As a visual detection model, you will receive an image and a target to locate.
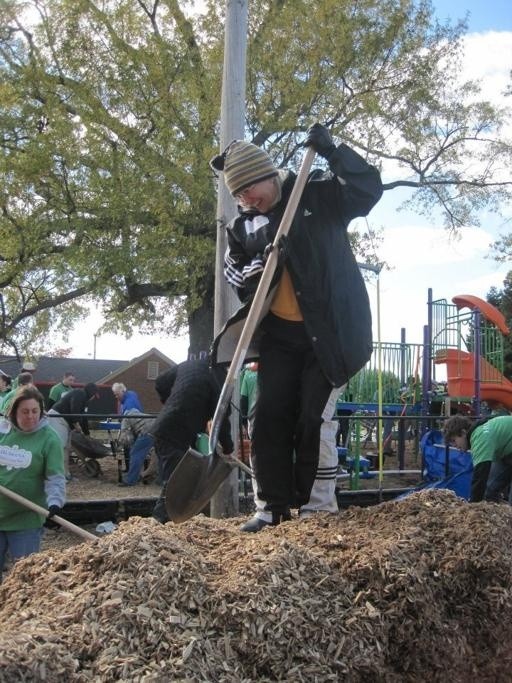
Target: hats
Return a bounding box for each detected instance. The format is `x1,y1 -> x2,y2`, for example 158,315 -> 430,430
209,139 -> 279,198
22,362 -> 36,370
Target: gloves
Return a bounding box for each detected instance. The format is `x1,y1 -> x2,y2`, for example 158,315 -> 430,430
303,123 -> 334,158
42,504 -> 63,531
263,233 -> 290,266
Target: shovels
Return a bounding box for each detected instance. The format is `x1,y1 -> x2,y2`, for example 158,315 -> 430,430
160,147 -> 319,525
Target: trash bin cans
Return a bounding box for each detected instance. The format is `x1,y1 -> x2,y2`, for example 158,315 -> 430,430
421,431 -> 473,500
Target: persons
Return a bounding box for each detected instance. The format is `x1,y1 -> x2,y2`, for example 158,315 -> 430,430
114,407 -> 161,488
1,382 -> 66,584
397,375 -> 415,403
238,359 -> 259,421
111,382 -> 143,475
440,412 -> 512,504
145,351 -> 236,527
45,381 -> 102,484
211,119 -> 384,532
1,371 -> 34,419
333,383 -> 353,448
1,370 -> 12,404
297,379 -> 347,517
45,372 -> 79,431
378,379 -> 398,456
12,361 -> 36,388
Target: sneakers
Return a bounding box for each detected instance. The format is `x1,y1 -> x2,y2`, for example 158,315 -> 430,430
240,504 -> 311,532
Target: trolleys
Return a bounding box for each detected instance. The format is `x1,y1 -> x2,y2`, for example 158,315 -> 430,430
70,430 -> 110,477
